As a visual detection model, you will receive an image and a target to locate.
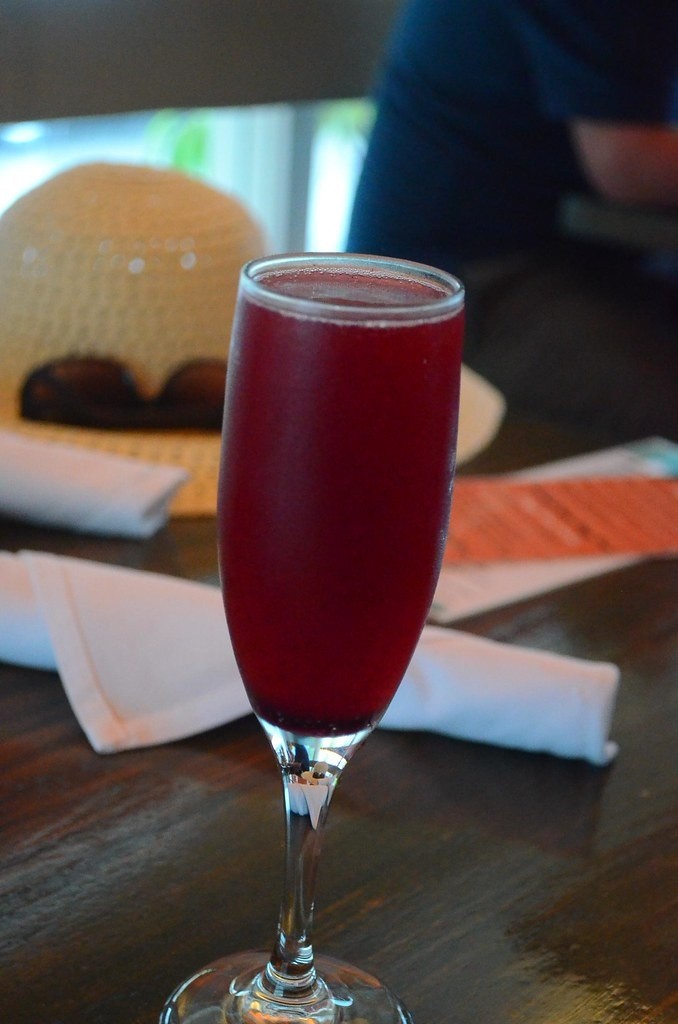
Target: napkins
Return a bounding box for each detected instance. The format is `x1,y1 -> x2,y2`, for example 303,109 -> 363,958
0,419 -> 678,1024
0,429 -> 190,539
0,549 -> 621,765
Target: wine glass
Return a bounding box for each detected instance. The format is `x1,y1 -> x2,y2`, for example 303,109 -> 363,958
155,251 -> 466,1024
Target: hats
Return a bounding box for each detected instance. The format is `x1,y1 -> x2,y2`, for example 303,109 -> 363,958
0,161 -> 507,518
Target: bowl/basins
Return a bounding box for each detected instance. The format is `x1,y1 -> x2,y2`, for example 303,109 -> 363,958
573,119 -> 678,208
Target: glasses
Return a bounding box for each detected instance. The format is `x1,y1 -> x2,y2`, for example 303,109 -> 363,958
20,353 -> 227,434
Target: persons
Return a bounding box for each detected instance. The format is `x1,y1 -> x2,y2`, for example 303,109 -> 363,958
346,0 -> 678,447
